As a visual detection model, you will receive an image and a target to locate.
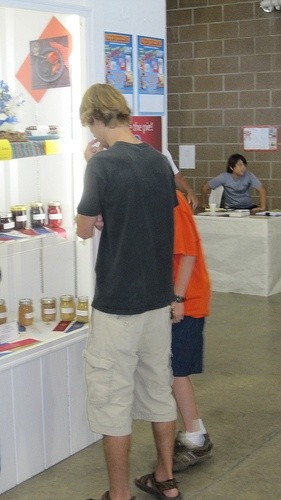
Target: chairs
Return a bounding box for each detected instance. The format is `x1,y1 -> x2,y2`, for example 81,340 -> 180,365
208,185 -> 224,208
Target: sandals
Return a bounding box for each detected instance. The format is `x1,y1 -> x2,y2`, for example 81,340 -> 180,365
135,473 -> 182,500
87,490 -> 137,500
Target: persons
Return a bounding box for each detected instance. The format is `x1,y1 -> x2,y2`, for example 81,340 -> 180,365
202,153 -> 266,212
167,149 -> 198,211
170,188 -> 215,474
75,83 -> 183,500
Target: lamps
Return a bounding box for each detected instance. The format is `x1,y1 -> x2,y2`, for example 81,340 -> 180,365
260,0 -> 281,13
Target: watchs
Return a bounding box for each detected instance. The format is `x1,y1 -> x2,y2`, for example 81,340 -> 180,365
174,295 -> 185,303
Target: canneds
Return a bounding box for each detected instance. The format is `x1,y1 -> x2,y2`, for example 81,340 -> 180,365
9,205 -> 28,229
60,295 -> 75,322
40,297 -> 57,321
30,201 -> 46,227
0,212 -> 15,232
19,298 -> 34,326
0,299 -> 7,324
76,296 -> 88,323
48,202 -> 62,228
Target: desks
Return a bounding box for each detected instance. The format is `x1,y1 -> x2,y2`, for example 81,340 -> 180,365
193,215 -> 281,297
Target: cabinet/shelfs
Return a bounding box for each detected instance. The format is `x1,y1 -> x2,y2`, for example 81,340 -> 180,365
0,139 -> 89,365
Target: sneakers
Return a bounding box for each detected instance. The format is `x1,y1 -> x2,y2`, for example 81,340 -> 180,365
171,432 -> 214,471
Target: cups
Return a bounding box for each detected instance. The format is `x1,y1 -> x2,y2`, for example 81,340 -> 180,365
209,204 -> 216,214
